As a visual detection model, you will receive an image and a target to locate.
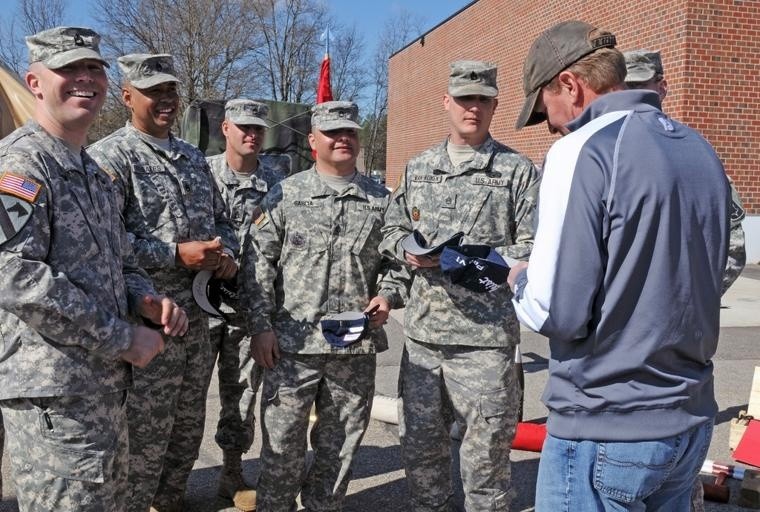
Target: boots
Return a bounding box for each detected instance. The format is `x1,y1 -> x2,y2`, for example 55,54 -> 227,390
215,446 -> 257,512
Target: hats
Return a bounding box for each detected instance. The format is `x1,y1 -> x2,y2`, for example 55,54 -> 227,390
114,52 -> 185,87
513,19 -> 618,130
401,230 -> 466,257
191,267 -> 241,319
309,100 -> 363,130
447,60 -> 501,99
440,243 -> 523,294
620,47 -> 665,82
224,98 -> 271,129
320,311 -> 371,347
23,25 -> 113,71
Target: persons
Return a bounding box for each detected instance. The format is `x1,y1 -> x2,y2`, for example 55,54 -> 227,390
0,25 -> 190,511
380,61 -> 540,511
241,100 -> 414,512
623,46 -> 747,511
81,50 -> 240,512
513,21 -> 732,511
203,98 -> 284,512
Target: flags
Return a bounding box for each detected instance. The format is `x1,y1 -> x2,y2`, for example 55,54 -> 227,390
309,58 -> 333,166
